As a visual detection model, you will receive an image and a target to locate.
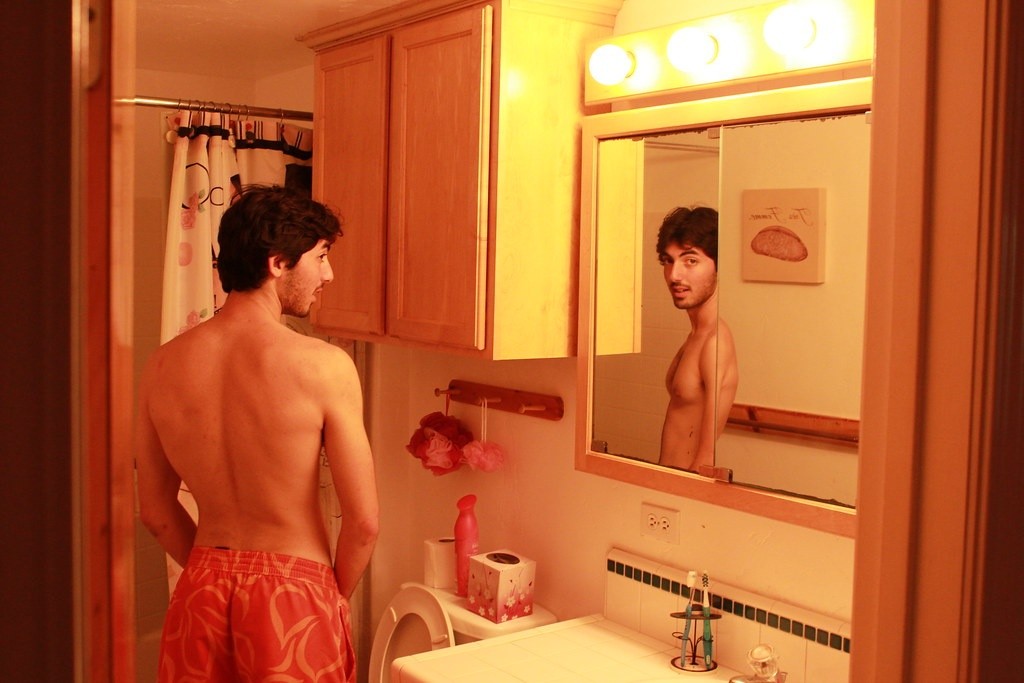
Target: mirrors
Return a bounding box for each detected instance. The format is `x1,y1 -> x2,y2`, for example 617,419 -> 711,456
568,102 -> 875,538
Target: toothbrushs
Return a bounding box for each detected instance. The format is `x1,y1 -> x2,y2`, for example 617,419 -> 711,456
680,570 -> 713,671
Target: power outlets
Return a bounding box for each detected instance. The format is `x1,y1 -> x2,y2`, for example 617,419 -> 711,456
637,501 -> 683,547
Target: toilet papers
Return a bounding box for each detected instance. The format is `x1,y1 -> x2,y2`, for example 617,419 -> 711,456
422,535 -> 460,590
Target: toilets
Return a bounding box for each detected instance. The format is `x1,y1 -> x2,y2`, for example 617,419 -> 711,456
275,584 -> 457,683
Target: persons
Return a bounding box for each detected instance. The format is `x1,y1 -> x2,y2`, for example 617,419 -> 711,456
656,205 -> 736,477
135,184 -> 379,683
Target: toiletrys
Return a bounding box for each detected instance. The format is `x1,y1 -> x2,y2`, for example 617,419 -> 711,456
453,494 -> 481,599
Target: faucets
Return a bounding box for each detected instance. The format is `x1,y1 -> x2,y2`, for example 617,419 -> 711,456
727,643 -> 787,683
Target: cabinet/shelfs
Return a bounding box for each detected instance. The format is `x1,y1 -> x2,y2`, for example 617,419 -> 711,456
434,379 -> 565,424
290,0 -> 578,362
